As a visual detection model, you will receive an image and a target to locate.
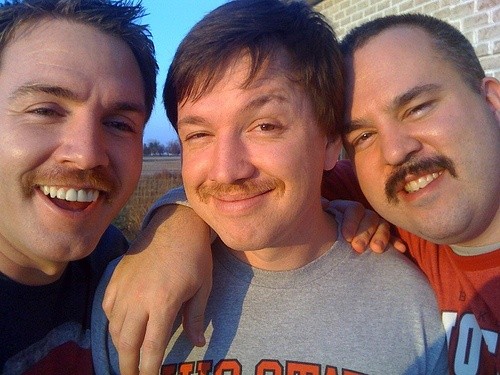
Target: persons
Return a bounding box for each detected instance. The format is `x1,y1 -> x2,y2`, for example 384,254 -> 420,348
0,0 -> 160,375
91,0 -> 452,374
101,12 -> 500,374
